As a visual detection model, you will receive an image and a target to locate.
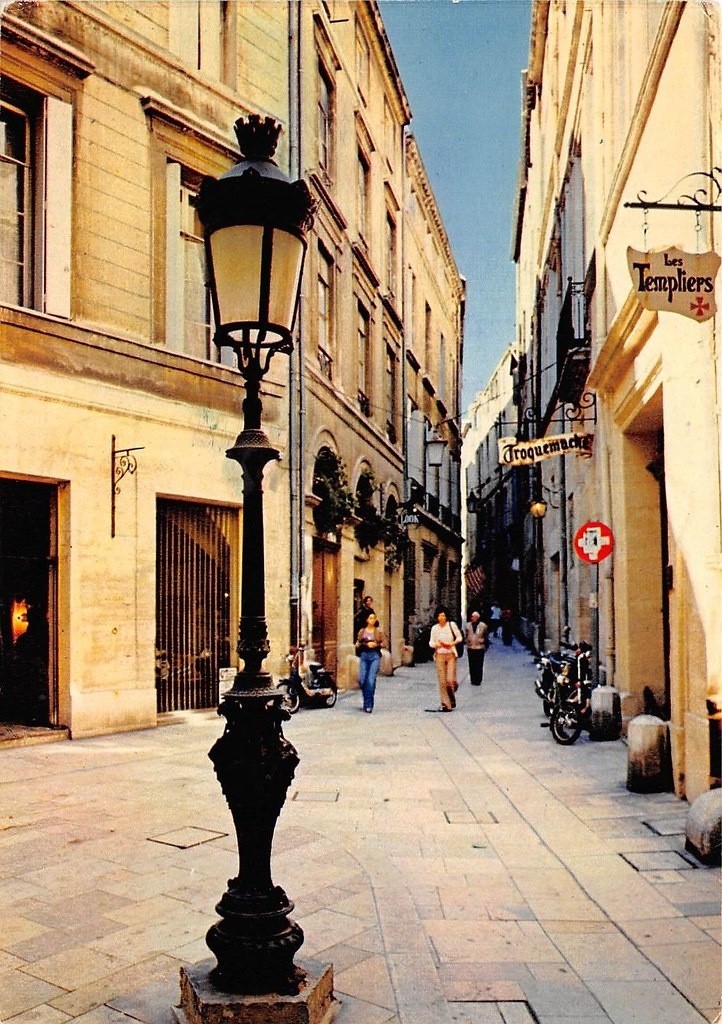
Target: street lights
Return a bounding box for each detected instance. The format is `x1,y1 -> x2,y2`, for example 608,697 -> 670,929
185,109 -> 322,997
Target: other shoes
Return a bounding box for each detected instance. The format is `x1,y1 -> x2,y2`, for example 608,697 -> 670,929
439,706 -> 452,712
366,708 -> 373,713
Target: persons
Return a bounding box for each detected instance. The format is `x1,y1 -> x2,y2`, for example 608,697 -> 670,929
476,599 -> 514,648
429,611 -> 463,712
355,595 -> 380,643
464,611 -> 488,687
355,612 -> 386,713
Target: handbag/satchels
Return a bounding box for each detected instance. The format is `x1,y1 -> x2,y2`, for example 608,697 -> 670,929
449,620 -> 464,658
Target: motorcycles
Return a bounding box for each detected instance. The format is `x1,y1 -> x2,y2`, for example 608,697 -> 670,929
533,622 -> 596,744
271,641 -> 338,715
